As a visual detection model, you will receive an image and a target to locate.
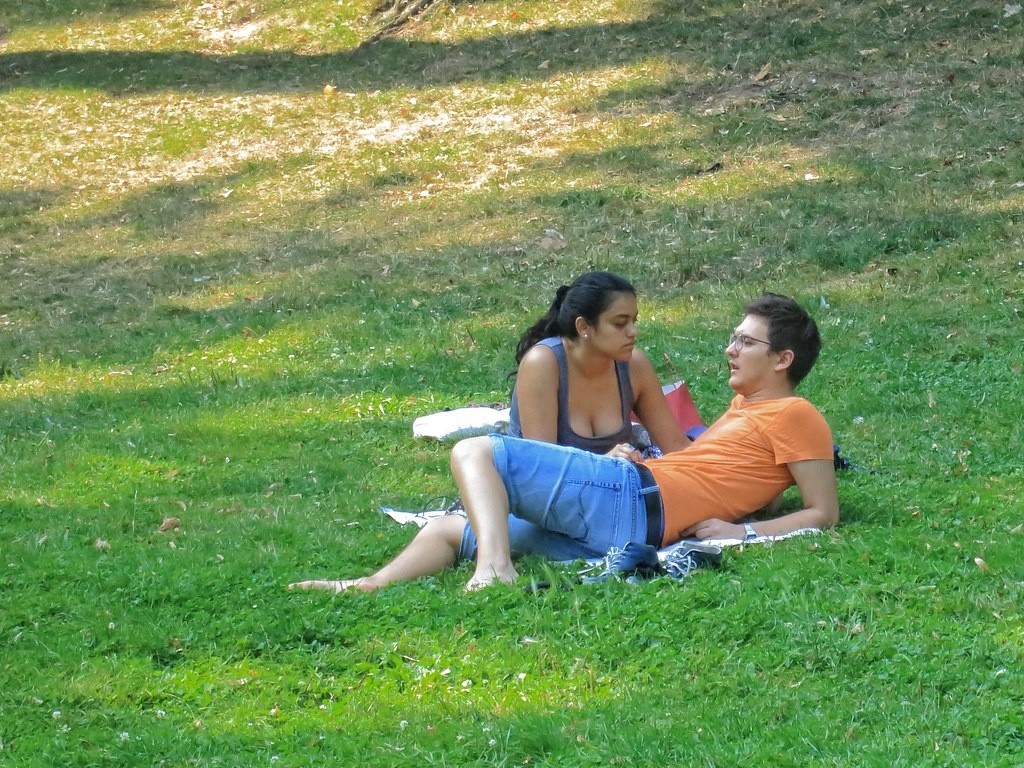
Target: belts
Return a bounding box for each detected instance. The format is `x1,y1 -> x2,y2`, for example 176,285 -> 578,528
630,461 -> 659,552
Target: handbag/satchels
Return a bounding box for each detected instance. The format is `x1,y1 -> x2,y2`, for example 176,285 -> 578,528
630,353 -> 704,432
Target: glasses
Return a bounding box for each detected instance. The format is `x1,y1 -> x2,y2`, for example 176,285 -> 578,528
729,330 -> 774,352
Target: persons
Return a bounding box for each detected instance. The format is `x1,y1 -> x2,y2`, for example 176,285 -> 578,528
509,272 -> 693,464
287,296 -> 840,596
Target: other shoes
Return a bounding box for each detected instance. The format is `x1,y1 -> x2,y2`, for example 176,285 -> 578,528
581,542 -> 658,592
626,541 -> 723,587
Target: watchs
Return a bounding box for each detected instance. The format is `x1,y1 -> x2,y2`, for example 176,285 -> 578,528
744,523 -> 758,541
